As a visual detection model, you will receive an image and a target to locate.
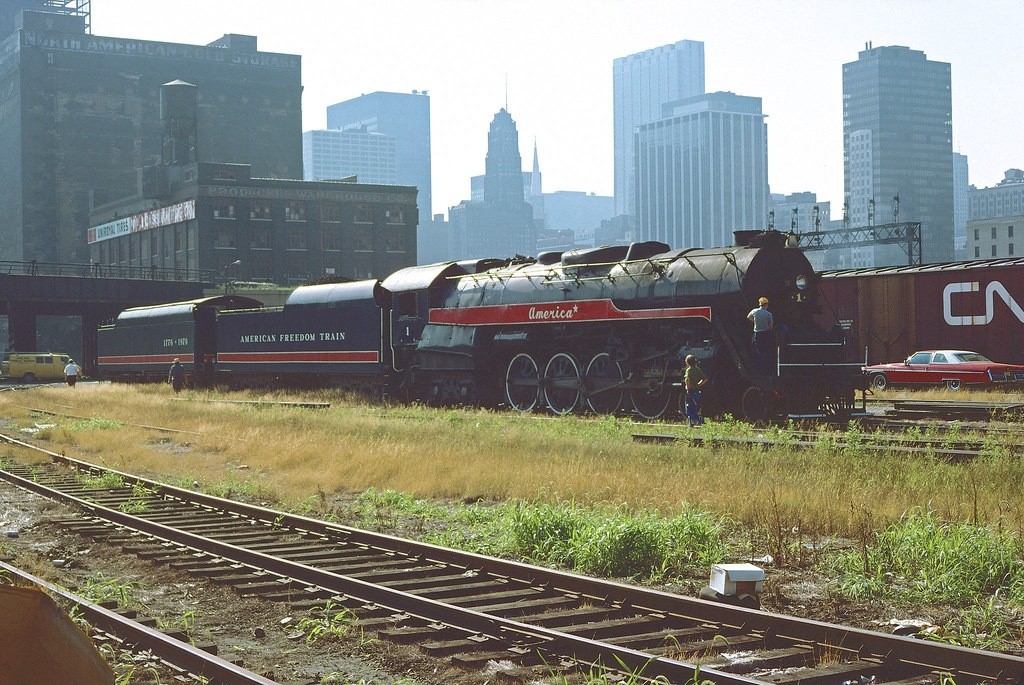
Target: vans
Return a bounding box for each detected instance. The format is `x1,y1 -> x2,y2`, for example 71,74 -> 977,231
9,352 -> 82,384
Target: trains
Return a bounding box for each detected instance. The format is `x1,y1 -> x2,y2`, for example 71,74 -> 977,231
98,231 -> 871,425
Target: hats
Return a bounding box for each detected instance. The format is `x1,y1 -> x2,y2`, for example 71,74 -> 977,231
759,297 -> 769,305
69,359 -> 73,361
174,358 -> 180,362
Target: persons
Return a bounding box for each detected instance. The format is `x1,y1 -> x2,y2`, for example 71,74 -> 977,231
685,355 -> 707,426
168,357 -> 185,392
64,359 -> 79,386
747,297 -> 774,362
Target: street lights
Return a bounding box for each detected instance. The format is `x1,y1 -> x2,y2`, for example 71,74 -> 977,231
225,260 -> 241,296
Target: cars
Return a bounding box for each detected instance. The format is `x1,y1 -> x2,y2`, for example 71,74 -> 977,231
861,350 -> 1024,392
